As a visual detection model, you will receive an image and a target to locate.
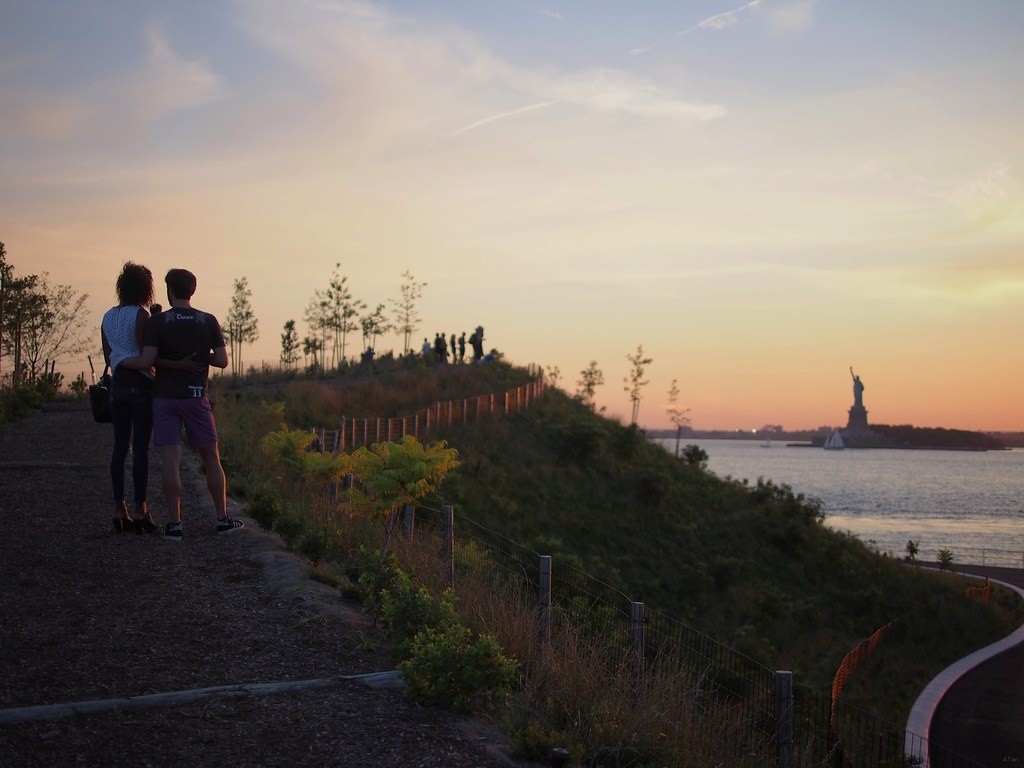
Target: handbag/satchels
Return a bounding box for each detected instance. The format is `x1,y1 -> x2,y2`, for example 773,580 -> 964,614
88,359 -> 113,423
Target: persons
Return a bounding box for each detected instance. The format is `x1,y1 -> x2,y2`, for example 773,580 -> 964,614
120,268 -> 244,542
100,262 -> 164,530
422,326 -> 496,366
849,366 -> 864,406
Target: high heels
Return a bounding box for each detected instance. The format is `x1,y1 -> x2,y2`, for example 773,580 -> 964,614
113,510 -> 134,534
133,511 -> 163,536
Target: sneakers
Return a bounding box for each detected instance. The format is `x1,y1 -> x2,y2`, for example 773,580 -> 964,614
158,521 -> 183,541
216,512 -> 245,536
